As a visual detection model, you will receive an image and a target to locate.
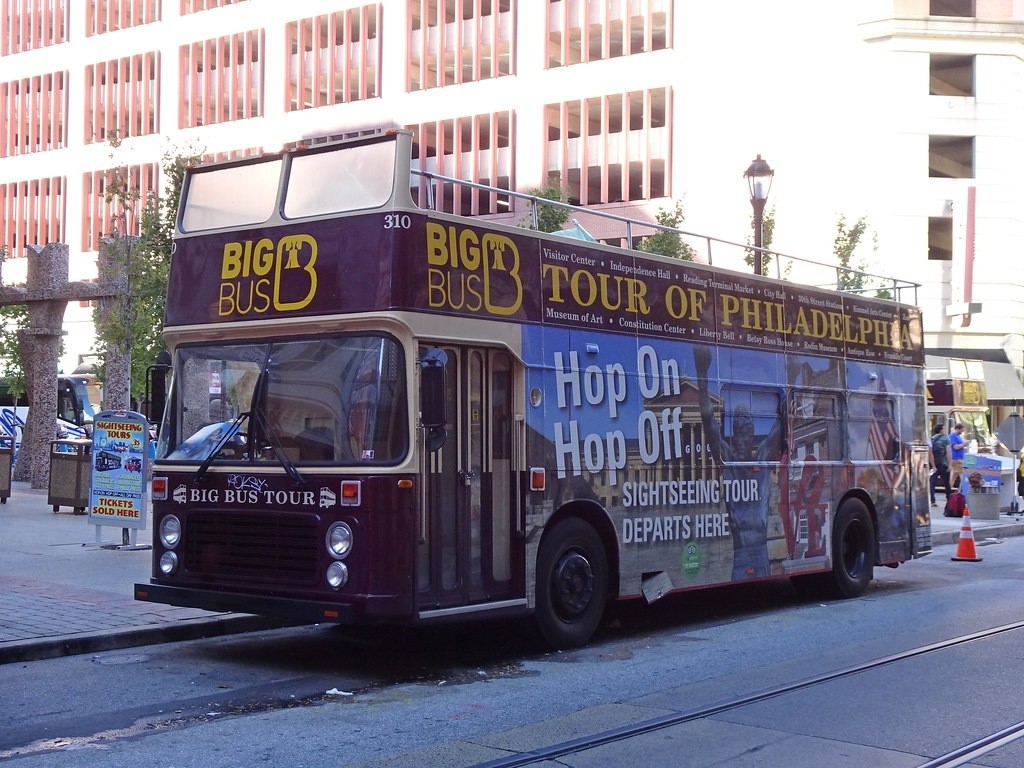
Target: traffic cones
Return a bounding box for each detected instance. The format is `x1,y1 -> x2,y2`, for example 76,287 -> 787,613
950,508 -> 983,562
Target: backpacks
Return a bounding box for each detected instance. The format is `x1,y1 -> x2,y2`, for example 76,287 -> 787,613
930,434 -> 947,467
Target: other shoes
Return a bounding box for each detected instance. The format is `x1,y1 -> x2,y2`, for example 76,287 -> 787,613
932,503 -> 937,507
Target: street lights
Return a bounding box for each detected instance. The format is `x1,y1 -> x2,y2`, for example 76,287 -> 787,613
744,155 -> 775,274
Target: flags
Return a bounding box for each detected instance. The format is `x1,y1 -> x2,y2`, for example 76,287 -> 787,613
869,372 -> 905,496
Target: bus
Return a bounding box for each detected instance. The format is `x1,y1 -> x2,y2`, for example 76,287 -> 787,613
131,122 -> 932,652
0,376 -> 96,460
924,358 -> 995,487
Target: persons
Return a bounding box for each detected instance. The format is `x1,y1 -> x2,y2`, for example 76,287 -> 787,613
930,424 -> 951,507
948,423 -> 970,486
1018,447 -> 1024,516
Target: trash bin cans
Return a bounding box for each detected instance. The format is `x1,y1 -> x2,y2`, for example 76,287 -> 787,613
963,453 -> 1001,520
48,438 -> 92,515
0,435 -> 16,504
